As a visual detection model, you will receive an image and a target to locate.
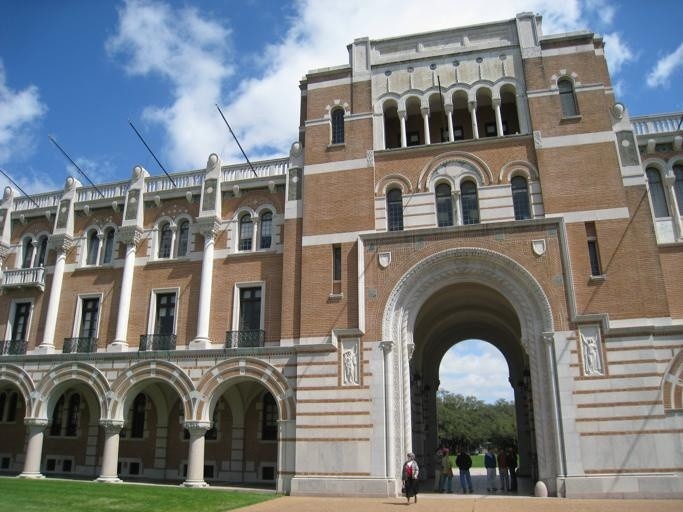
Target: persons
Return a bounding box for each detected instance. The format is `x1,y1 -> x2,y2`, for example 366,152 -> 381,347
432,446 -> 518,493
402,452 -> 420,504
